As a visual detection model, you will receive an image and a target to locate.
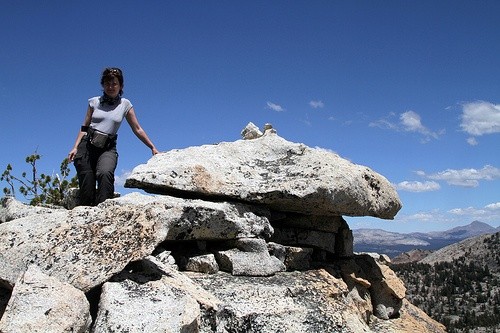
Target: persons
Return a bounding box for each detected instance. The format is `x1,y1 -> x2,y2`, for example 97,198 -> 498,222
68,68 -> 160,206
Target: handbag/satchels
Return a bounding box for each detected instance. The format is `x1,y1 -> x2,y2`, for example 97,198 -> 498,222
81,126 -> 117,149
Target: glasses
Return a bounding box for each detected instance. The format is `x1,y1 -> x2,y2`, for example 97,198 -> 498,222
103,70 -> 121,76
